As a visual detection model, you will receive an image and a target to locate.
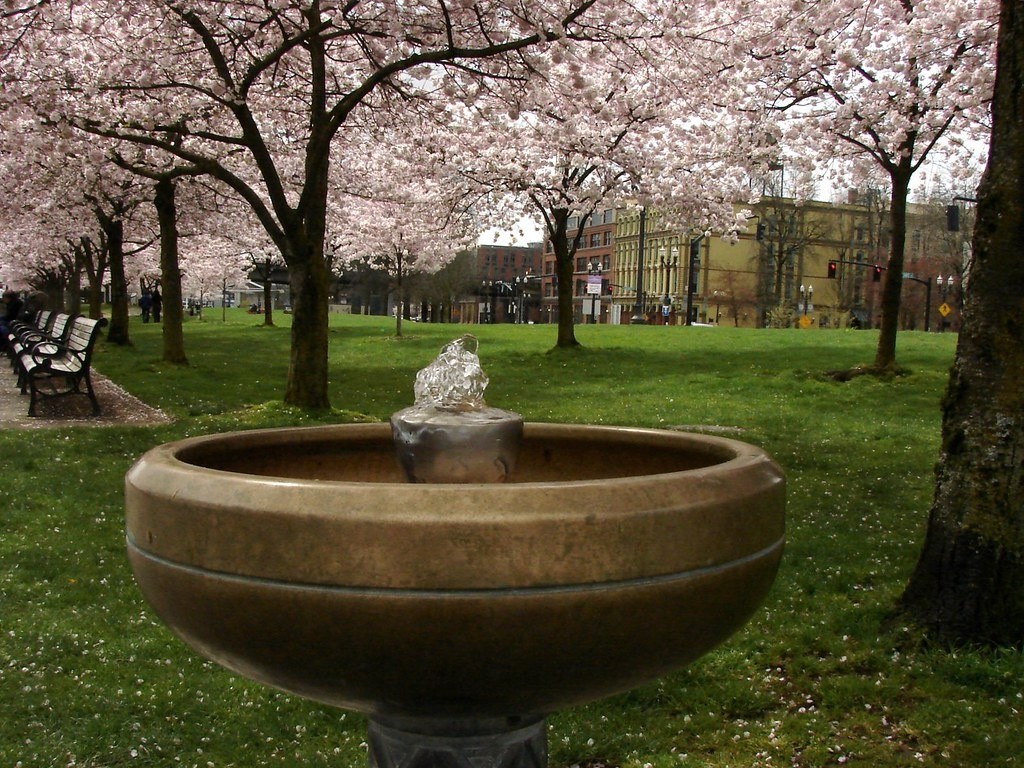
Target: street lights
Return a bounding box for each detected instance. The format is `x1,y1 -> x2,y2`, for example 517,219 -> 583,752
515,275 -> 528,324
937,273 -> 954,334
800,284 -> 814,316
587,261 -> 603,323
769,162 -> 784,306
714,290 -> 725,327
659,244 -> 679,326
482,280 -> 492,324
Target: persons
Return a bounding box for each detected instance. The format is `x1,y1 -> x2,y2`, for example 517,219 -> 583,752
0,291 -> 23,333
138,289 -> 162,323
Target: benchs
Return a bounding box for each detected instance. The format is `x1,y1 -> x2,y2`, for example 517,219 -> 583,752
5,309 -> 111,420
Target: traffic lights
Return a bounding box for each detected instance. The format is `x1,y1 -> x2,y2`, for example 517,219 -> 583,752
827,262 -> 836,279
872,265 -> 882,283
607,286 -> 612,295
755,223 -> 767,241
